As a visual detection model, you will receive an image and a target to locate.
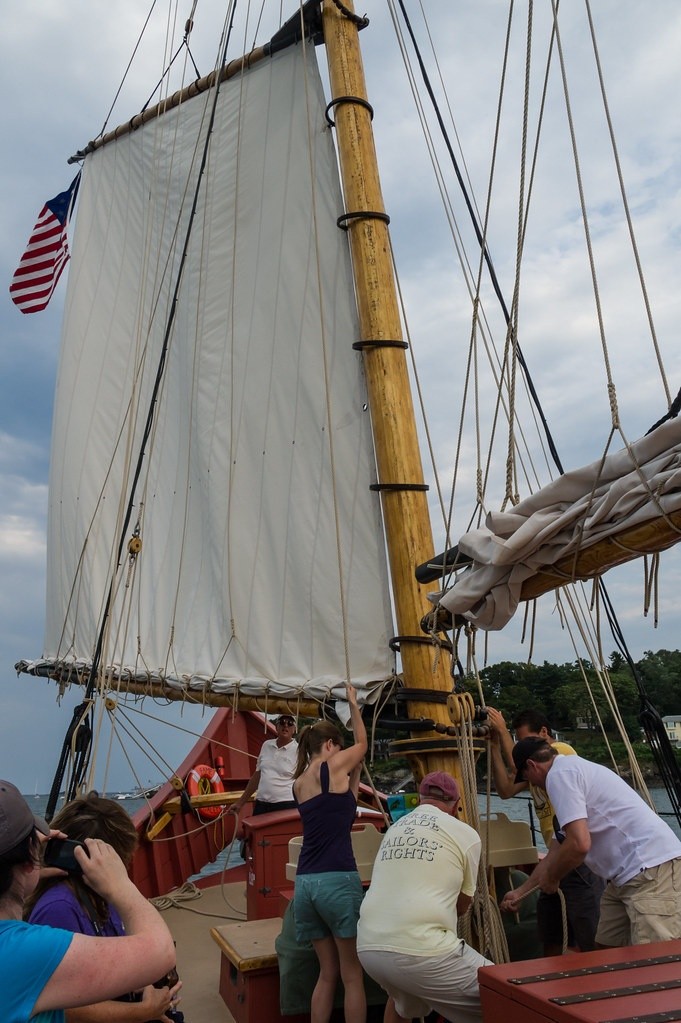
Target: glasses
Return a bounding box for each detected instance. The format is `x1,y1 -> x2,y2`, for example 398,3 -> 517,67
278,719 -> 295,726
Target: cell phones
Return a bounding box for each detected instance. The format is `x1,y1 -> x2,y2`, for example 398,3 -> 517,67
42,837 -> 94,876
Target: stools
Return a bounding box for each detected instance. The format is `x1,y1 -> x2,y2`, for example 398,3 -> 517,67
210,915 -> 317,1022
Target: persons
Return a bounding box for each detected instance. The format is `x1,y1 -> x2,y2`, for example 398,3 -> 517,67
23,790 -> 177,1022
357,771 -> 497,1023
511,734 -> 678,950
227,715 -> 299,900
479,704 -> 605,960
288,681 -> 371,1023
0,781 -> 176,1022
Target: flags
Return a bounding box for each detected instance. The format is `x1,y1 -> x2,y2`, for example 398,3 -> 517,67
7,167 -> 81,312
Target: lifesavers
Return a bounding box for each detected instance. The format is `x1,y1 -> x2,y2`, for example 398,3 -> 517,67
187,765 -> 225,819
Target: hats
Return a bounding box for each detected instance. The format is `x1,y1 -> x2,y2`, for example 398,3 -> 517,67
277,714 -> 297,722
0,779 -> 50,856
420,772 -> 459,801
513,736 -> 548,785
